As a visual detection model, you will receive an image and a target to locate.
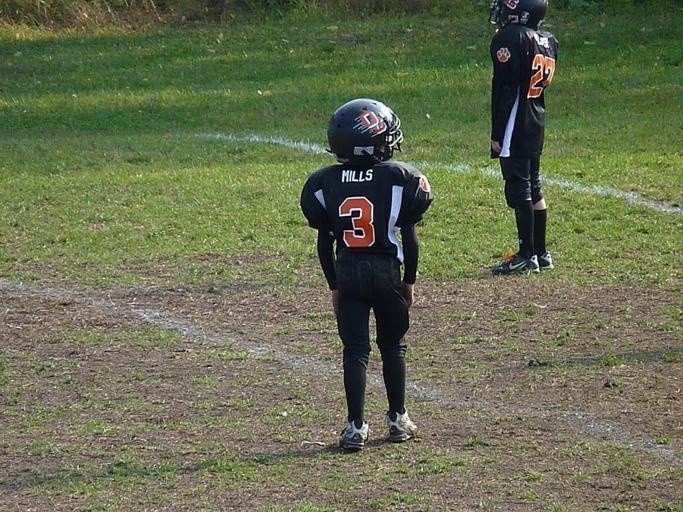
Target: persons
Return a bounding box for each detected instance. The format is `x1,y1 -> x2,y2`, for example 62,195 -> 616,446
299,98 -> 437,450
486,1 -> 560,274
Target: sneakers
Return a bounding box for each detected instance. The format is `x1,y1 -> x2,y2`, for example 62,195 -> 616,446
492,253 -> 540,275
538,251 -> 555,269
339,419 -> 370,450
387,407 -> 418,442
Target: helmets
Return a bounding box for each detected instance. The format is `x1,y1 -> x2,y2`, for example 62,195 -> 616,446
327,99 -> 393,163
494,0 -> 549,28
